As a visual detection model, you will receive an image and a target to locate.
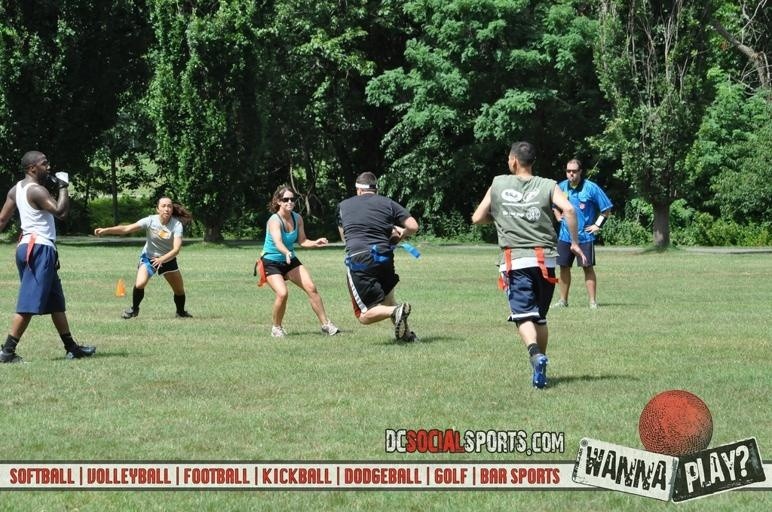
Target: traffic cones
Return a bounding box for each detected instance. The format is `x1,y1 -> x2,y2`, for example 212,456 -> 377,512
113,277 -> 129,298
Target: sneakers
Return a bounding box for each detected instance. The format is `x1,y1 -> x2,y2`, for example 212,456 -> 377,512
530,353 -> 547,389
272,326 -> 288,337
549,301 -> 568,309
322,323 -> 340,336
590,303 -> 598,310
175,312 -> 193,318
66,346 -> 96,359
121,307 -> 139,318
392,303 -> 416,343
0,350 -> 23,363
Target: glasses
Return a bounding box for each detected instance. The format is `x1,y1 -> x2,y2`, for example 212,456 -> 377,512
281,198 -> 296,202
567,170 -> 577,173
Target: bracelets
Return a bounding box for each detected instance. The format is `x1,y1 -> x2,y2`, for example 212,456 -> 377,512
595,214 -> 607,228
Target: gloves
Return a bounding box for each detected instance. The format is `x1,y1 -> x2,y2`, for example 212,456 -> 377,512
50,172 -> 69,189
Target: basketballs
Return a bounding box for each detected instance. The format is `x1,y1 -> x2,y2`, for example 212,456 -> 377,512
639,390 -> 713,457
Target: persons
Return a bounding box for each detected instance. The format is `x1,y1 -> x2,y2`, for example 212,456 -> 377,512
337,172 -> 419,343
254,185 -> 339,337
0,151 -> 96,361
472,143 -> 587,389
552,159 -> 613,308
94,196 -> 193,318
